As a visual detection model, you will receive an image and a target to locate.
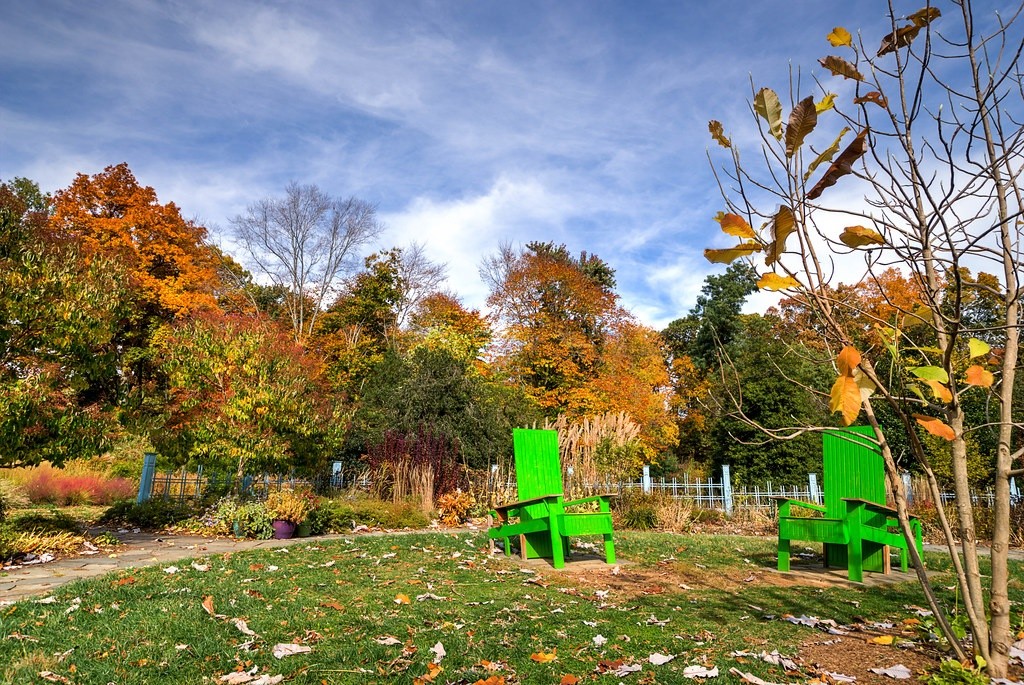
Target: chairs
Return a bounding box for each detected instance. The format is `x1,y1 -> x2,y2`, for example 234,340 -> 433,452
769,425 -> 924,583
487,428 -> 619,569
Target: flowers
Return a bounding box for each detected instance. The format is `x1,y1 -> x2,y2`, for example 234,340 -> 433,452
266,485 -> 321,526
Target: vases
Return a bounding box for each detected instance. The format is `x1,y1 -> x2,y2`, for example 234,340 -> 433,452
271,520 -> 298,541
293,518 -> 313,538
